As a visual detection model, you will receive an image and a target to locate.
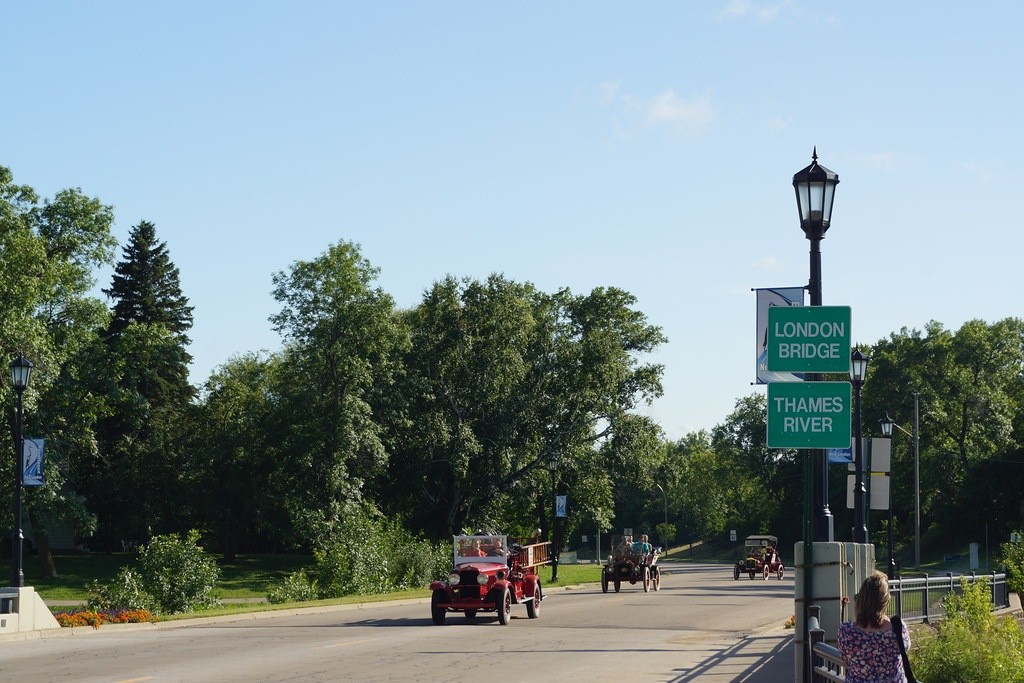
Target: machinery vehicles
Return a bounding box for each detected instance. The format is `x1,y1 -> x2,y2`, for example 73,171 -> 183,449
428,529 -> 552,625
600,534 -> 662,593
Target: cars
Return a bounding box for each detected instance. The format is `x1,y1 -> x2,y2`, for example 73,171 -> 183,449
732,535 -> 786,581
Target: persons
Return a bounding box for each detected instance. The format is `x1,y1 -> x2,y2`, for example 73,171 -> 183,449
836,571 -> 911,683
487,538 -> 509,556
643,534 -> 652,553
750,542 -> 777,564
633,535 -> 649,555
627,535 -> 635,545
464,539 -> 486,558
616,535 -> 633,555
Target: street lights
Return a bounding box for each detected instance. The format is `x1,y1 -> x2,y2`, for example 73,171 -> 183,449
791,146 -> 844,683
656,482 -> 667,560
877,412 -> 896,596
547,455 -> 561,583
846,340 -> 872,545
6,350 -> 34,589
